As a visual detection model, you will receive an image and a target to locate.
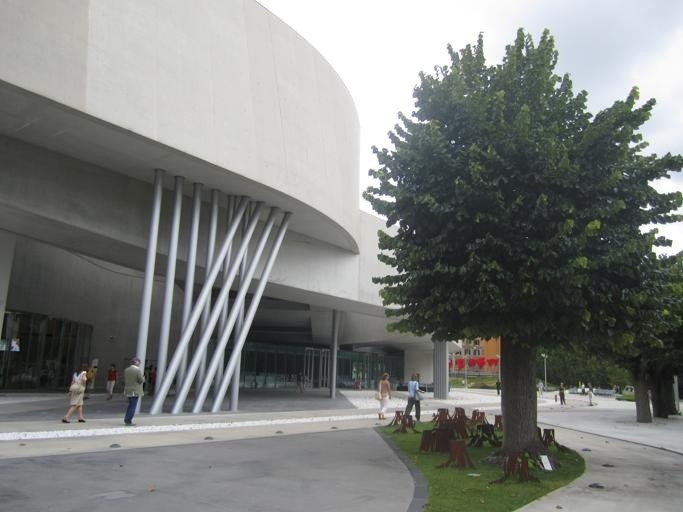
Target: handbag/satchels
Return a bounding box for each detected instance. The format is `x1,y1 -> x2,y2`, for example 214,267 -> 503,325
69,383 -> 84,392
415,392 -> 424,401
375,392 -> 381,399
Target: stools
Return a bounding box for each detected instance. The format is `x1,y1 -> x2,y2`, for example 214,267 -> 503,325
383,405 -> 562,484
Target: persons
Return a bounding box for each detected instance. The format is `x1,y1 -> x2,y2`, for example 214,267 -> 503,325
588,389 -> 593,406
105,364 -> 116,400
496,379 -> 501,394
376,373 -> 392,419
559,382 -> 567,407
62,364 -> 89,423
403,374 -> 420,421
578,381 -> 592,394
295,371 -> 304,393
537,379 -> 544,398
123,357 -> 145,425
613,384 -> 621,394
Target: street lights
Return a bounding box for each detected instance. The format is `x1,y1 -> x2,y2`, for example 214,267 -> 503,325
464,343 -> 479,390
540,352 -> 548,390
